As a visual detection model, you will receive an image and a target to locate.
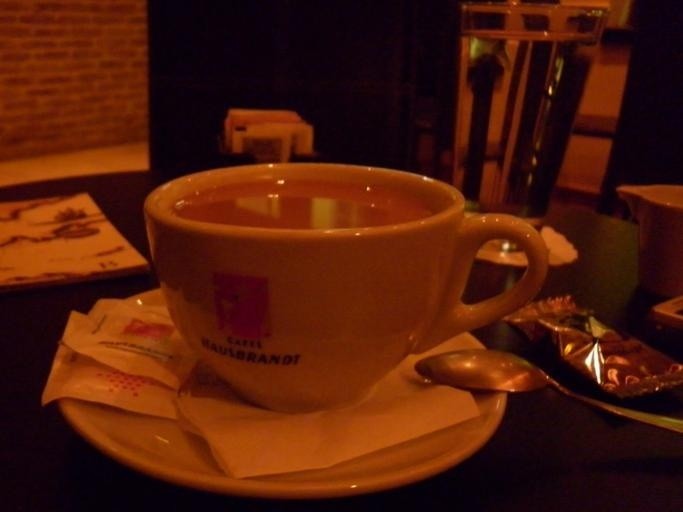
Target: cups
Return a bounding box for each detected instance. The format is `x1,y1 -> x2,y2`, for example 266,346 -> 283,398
617,183 -> 683,303
140,161 -> 548,404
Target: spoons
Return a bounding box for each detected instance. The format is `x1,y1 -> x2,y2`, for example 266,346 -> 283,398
414,349 -> 682,434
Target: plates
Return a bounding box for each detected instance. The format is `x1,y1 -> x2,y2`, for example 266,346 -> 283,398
63,287 -> 506,494
